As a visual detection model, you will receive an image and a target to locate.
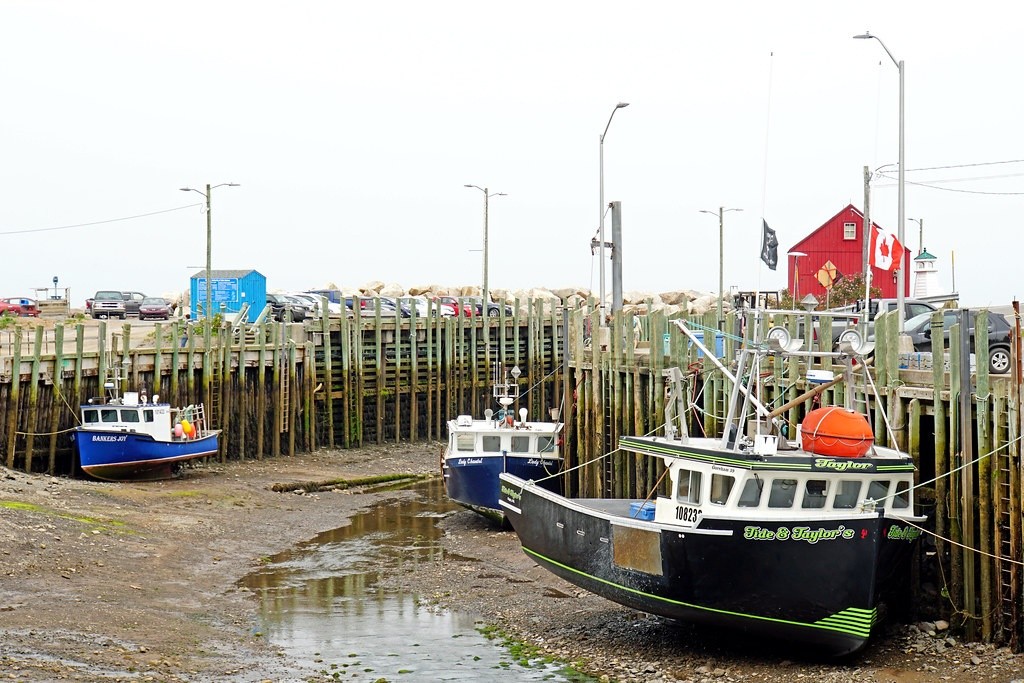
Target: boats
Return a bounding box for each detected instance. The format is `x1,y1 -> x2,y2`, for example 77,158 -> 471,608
439,360 -> 569,529
498,317 -> 934,671
61,355 -> 224,483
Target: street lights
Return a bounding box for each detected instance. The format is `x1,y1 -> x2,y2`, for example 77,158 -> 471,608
699,206 -> 745,317
178,181 -> 242,330
598,100 -> 630,325
852,30 -> 908,345
463,181 -> 507,313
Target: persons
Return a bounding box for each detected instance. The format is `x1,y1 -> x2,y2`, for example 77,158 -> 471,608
606,310 -> 642,350
180,314 -> 193,347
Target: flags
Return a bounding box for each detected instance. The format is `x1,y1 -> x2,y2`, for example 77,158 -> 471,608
760,218 -> 779,271
868,224 -> 904,271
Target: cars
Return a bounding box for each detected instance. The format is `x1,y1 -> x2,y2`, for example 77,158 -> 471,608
90,290 -> 127,320
137,297 -> 171,321
122,291 -> 152,314
283,289 -> 480,319
265,294 -> 306,323
460,296 -> 513,318
0,297 -> 41,318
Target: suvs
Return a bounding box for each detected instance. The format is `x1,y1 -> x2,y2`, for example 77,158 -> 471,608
866,308 -> 1013,375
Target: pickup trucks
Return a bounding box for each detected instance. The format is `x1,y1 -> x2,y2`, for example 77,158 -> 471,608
806,293 -> 937,366
